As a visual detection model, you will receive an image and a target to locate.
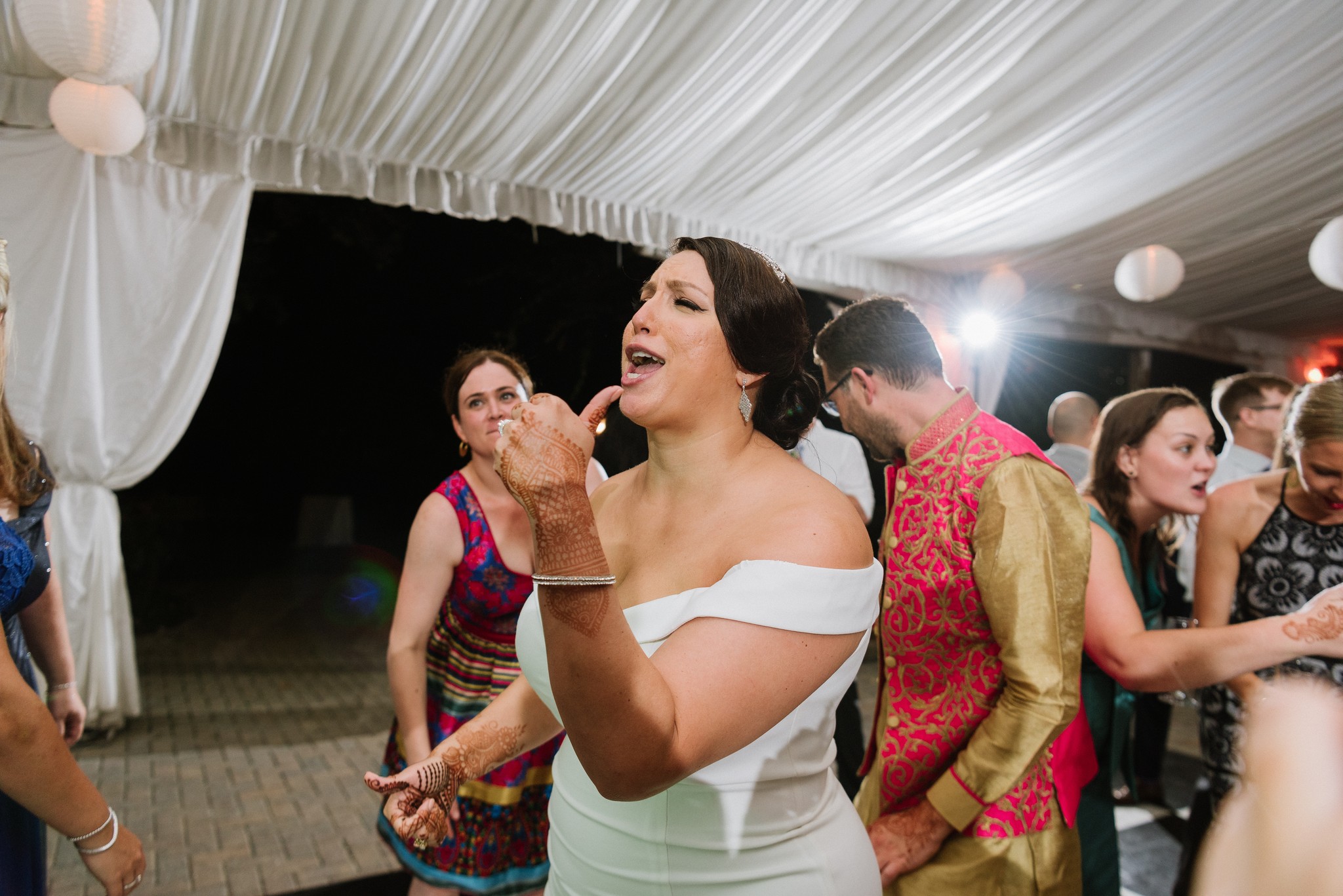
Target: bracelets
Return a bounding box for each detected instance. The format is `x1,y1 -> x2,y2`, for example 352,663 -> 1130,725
529,574 -> 617,586
67,806 -> 114,842
78,811 -> 119,855
47,681 -> 78,692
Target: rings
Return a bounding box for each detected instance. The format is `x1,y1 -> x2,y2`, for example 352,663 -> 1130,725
413,836 -> 428,852
122,874 -> 142,890
498,417 -> 515,436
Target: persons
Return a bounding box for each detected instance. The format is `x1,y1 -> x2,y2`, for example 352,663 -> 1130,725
744,290 -> 1342,895
0,236 -> 86,896
374,345 -> 610,896
0,512 -> 150,896
362,236 -> 886,896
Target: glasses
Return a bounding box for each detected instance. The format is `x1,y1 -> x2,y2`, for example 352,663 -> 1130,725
820,368 -> 874,417
